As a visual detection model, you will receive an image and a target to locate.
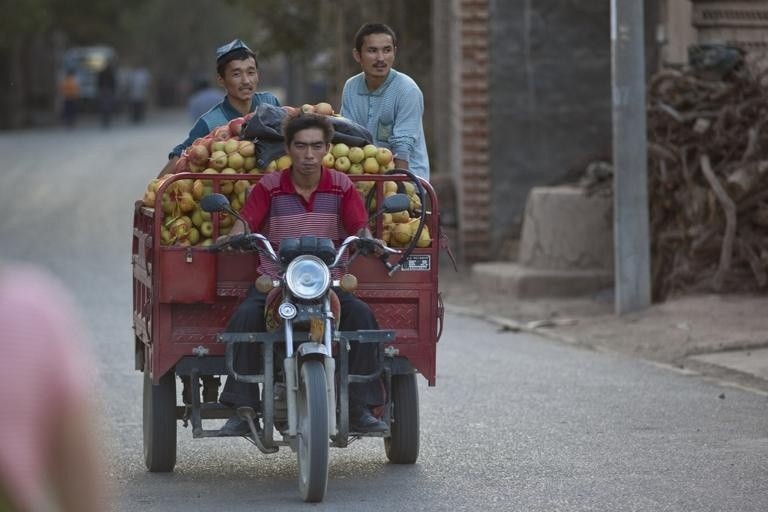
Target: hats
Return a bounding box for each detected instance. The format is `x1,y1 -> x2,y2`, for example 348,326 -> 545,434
216,39 -> 253,60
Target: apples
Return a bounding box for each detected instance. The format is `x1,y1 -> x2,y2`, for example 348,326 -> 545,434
143,102 -> 431,247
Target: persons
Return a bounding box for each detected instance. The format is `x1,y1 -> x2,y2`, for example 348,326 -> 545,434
217,112 -> 390,435
340,21 -> 430,202
55,52 -> 148,130
156,37 -> 284,179
0,264 -> 112,511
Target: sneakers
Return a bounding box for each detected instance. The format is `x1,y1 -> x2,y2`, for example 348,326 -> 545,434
220,415 -> 259,435
350,406 -> 388,432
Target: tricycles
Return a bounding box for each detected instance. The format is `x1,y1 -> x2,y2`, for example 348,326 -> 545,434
128,169 -> 446,506
